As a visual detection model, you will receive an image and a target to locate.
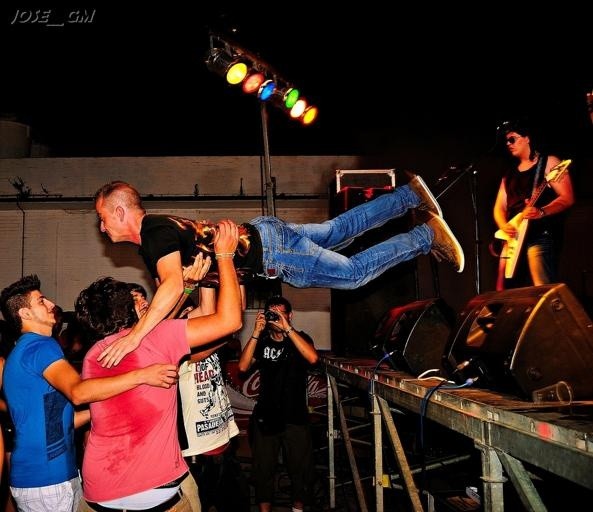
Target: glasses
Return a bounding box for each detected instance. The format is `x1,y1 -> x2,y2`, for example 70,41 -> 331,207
507,135 -> 521,143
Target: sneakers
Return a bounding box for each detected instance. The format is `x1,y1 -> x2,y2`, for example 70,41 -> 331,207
409,175 -> 443,218
428,217 -> 466,273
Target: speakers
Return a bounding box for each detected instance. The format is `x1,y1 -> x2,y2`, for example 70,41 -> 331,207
331,189 -> 420,360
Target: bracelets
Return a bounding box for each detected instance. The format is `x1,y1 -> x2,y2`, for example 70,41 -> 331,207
285,327 -> 293,335
251,335 -> 260,340
537,206 -> 545,217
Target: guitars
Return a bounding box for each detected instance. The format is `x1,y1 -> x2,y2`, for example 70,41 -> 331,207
495,159 -> 570,280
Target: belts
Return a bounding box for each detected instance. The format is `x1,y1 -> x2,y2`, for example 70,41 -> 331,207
87,488 -> 182,512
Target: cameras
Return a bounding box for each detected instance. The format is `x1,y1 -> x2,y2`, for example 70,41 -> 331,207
264,311 -> 279,322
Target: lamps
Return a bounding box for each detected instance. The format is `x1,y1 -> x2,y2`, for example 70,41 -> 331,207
202,36 -> 319,127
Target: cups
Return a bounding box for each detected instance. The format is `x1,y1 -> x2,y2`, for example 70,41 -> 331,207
532,380 -> 573,406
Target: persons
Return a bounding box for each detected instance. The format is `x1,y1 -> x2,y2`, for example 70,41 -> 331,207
238,295 -> 319,511
0,251 -> 247,512
492,118 -> 575,288
93,172 -> 467,371
73,218 -> 245,512
